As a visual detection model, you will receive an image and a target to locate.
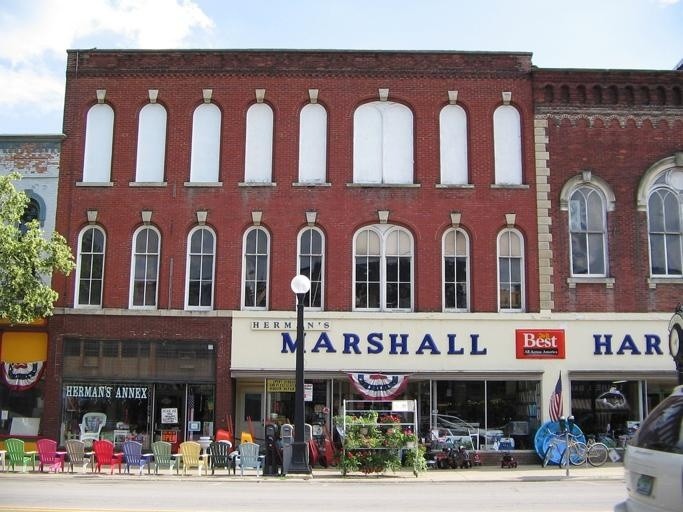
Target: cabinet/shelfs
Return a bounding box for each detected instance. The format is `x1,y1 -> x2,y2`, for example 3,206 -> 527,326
334,400 -> 420,478
514,381 -> 540,449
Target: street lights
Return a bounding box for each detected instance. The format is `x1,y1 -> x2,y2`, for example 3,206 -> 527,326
287,275 -> 311,474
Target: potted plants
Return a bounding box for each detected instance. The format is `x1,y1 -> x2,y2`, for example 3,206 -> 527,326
333,412 -> 428,479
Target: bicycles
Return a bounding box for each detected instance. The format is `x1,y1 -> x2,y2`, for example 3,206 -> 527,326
559,433 -> 608,469
541,424 -> 588,468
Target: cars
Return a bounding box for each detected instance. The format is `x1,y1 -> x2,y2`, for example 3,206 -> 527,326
419,413 -> 504,451
614,385 -> 682,511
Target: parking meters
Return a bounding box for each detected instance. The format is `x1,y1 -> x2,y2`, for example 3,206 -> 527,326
558,414 -> 574,477
667,303 -> 682,386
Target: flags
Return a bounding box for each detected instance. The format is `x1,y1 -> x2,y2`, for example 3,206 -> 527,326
549,376 -> 562,422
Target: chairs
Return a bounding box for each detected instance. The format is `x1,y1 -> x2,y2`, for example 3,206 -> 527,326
0,434 -> 265,478
77,412 -> 107,442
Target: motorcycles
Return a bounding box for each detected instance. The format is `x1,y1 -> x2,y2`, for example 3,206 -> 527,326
421,438 -> 481,469
